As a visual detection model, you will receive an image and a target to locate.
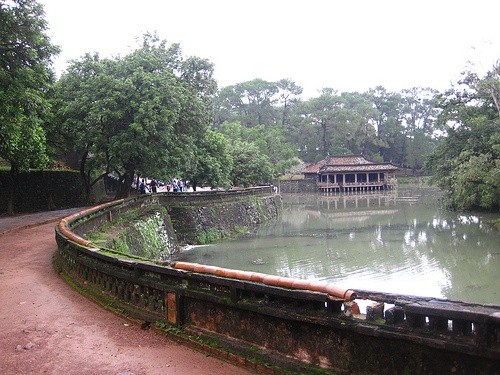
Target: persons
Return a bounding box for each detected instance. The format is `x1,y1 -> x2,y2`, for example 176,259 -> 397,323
140,178 -> 190,193
